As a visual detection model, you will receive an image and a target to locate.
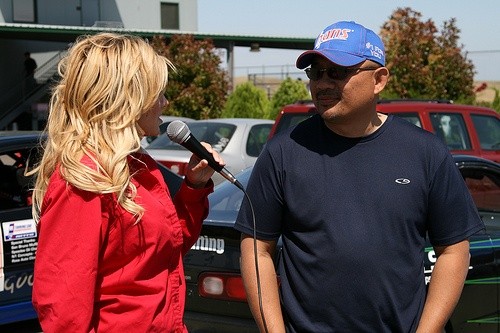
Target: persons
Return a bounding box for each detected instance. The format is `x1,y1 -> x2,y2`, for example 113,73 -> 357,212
232,21 -> 484,333
24,31 -> 226,333
21,51 -> 37,93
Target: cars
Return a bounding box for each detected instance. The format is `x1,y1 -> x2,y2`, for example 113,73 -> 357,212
0,129 -> 51,326
140,118 -> 275,186
182,155 -> 500,333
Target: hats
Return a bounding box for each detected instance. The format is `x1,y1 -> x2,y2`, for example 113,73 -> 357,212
296,20 -> 385,69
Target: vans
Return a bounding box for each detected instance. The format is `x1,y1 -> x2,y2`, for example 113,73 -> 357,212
264,97 -> 500,212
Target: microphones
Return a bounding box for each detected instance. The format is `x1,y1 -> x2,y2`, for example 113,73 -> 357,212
167,120 -> 244,191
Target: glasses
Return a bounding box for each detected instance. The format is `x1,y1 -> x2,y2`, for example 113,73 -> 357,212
304,67 -> 376,82
